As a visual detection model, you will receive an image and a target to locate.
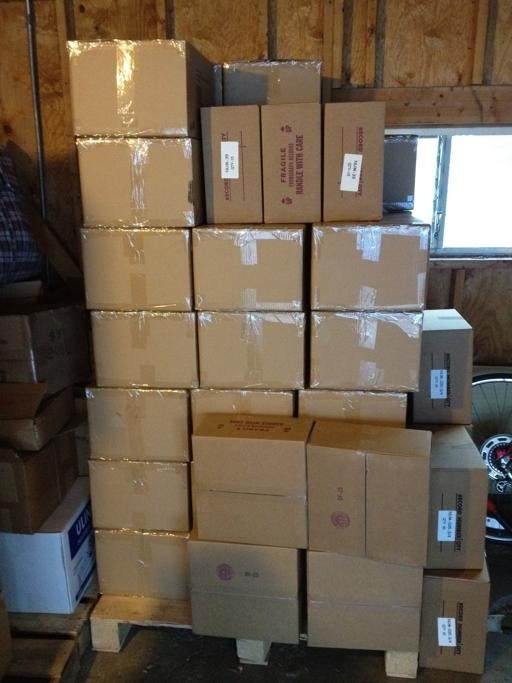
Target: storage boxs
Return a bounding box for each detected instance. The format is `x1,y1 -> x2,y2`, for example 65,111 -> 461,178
1,33 -> 493,675
